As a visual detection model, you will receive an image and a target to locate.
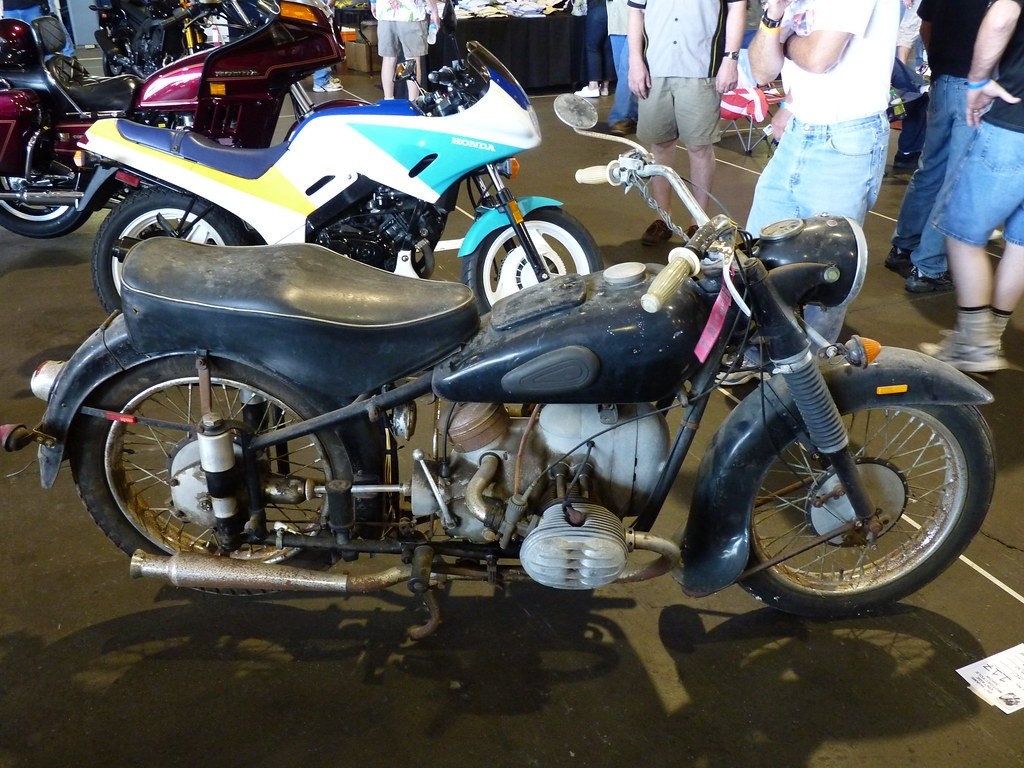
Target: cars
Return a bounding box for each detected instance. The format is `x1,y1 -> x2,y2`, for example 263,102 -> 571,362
0,0 -> 76,92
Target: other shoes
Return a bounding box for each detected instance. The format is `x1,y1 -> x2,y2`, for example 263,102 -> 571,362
611,120 -> 632,135
641,220 -> 673,245
683,225 -> 699,237
893,152 -> 921,167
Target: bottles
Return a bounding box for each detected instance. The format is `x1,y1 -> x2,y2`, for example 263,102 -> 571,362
890,90 -> 907,119
427,22 -> 437,45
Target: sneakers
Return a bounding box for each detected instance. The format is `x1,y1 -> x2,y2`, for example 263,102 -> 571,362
919,335 -> 999,372
574,86 -> 599,97
905,265 -> 953,293
885,247 -> 914,274
313,80 -> 343,92
599,87 -> 609,96
329,76 -> 340,84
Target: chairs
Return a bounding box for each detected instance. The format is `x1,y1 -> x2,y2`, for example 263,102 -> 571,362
720,30 -> 785,156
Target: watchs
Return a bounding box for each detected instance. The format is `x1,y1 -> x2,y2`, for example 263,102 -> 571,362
724,51 -> 739,60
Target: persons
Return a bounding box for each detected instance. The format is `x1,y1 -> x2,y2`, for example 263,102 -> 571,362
2,0 -> 76,62
370,0 -> 463,99
716,0 -> 900,386
573,0 -> 748,248
313,0 -> 343,93
883,0 -> 1024,382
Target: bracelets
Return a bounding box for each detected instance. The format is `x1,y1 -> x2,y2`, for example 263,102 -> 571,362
968,78 -> 990,89
761,8 -> 784,28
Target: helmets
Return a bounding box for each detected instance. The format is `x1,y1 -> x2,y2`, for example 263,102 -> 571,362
0,19 -> 40,69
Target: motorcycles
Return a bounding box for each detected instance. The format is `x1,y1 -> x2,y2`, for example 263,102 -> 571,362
0,0 -> 374,239
0,93 -> 998,621
73,0 -> 606,317
